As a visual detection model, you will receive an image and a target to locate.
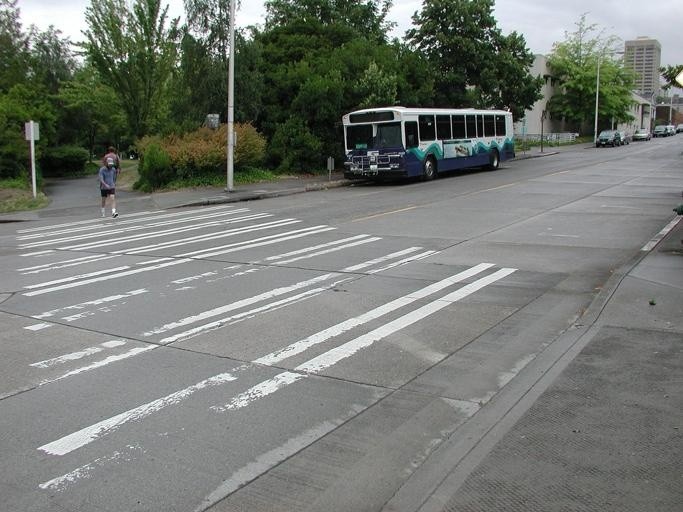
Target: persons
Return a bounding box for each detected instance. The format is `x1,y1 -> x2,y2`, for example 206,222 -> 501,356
102,145 -> 122,187
98,158 -> 118,218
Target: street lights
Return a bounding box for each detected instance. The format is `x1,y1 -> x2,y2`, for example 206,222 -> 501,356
593,48 -> 626,149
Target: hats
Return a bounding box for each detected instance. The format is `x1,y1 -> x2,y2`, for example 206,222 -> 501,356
107,158 -> 113,164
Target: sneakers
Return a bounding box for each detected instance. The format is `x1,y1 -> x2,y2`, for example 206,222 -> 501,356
101,212 -> 118,218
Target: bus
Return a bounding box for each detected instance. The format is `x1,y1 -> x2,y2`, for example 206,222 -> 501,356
341,104 -> 517,183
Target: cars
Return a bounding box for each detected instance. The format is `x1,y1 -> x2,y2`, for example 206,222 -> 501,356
594,124 -> 682,148
